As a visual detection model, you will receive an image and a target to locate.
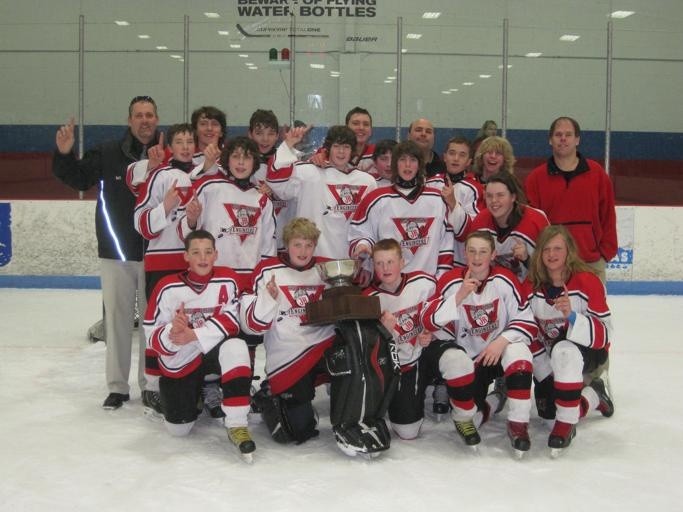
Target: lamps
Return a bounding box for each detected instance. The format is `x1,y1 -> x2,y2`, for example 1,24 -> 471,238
269,47 -> 289,62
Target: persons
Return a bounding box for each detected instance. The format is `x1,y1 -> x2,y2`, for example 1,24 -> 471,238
51,92 -> 623,463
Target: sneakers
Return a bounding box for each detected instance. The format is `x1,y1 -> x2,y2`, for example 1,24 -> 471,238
433,384 -> 448,414
506,421 -> 530,451
452,419 -> 480,445
103,393 -> 129,409
548,421 -> 576,448
143,389 -> 162,415
226,426 -> 255,453
590,378 -> 613,417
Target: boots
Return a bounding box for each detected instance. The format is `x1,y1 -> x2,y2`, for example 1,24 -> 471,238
203,380 -> 225,417
